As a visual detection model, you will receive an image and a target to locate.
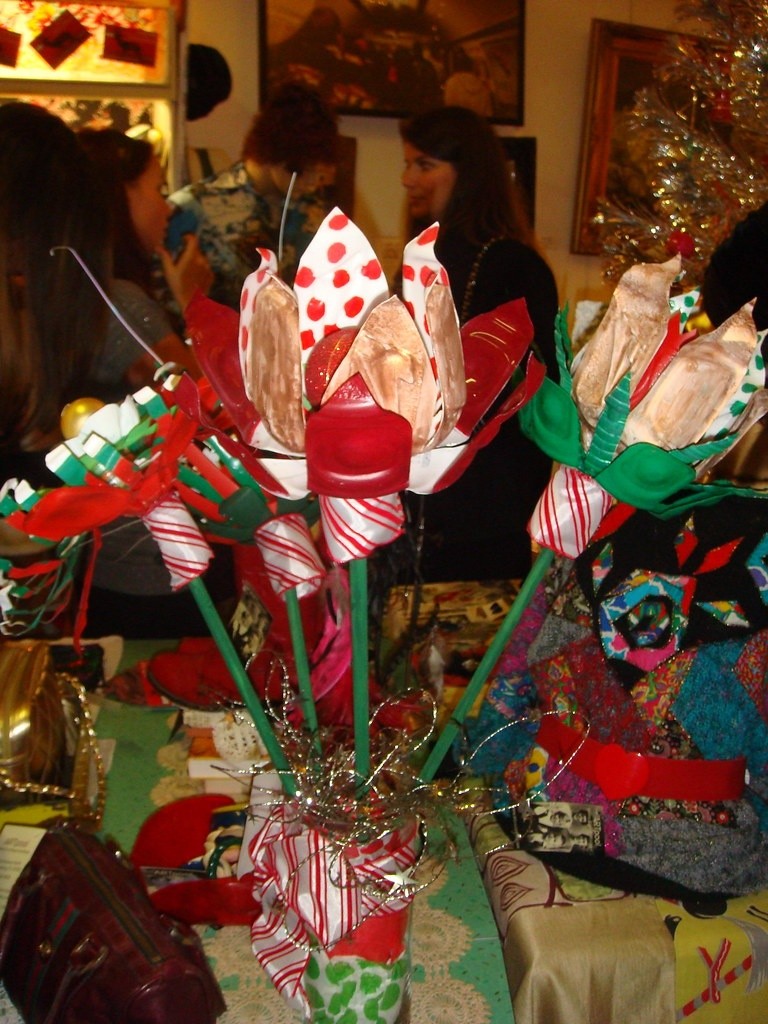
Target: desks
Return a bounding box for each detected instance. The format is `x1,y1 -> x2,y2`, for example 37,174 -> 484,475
374,581 -> 768,1024
4,634 -> 518,1024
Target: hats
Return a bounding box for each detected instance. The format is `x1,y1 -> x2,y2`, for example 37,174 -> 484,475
243,79 -> 350,165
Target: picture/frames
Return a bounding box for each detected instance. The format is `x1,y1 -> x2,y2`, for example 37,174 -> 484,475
567,13 -> 734,258
405,136 -> 537,249
259,0 -> 526,126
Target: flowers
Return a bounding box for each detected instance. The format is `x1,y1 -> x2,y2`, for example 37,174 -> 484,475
2,183 -> 768,845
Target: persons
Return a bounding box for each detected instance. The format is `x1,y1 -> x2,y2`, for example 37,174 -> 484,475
372,106 -> 567,580
152,100 -> 344,340
1,100 -> 237,639
702,200 -> 768,477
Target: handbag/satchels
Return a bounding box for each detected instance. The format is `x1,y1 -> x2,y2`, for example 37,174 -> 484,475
0,815 -> 229,1024
